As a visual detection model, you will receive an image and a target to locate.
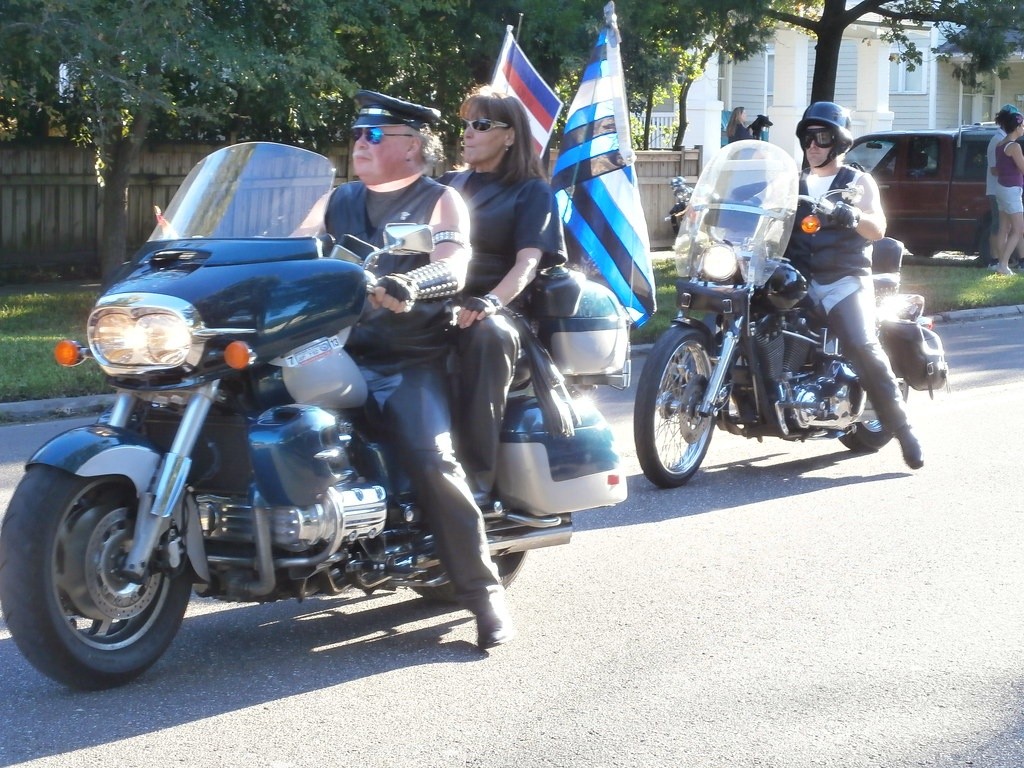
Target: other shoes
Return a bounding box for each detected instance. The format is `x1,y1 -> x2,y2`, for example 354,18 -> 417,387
1018,262 -> 1024,271
987,259 -> 999,270
892,424 -> 925,469
470,594 -> 516,649
995,271 -> 1017,276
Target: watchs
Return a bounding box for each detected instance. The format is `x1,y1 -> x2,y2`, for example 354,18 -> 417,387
853,207 -> 859,228
485,293 -> 501,308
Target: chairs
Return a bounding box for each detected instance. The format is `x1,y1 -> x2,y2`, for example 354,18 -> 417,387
909,153 -> 929,177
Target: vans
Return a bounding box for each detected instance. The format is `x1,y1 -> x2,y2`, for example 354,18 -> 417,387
841,122 -> 1023,265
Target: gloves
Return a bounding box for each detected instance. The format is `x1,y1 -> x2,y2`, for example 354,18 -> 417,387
826,200 -> 855,230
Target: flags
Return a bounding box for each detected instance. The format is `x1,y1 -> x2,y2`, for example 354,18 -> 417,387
551,1 -> 657,327
487,24 -> 562,162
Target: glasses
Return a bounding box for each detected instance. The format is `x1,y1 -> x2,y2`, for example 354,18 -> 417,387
352,128 -> 412,144
462,118 -> 512,133
799,127 -> 838,149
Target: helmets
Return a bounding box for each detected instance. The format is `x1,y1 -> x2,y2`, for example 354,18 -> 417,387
795,101 -> 854,154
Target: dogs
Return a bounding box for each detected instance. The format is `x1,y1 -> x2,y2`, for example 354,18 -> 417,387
746,114 -> 773,140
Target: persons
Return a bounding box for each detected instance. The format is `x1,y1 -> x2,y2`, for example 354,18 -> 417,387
724,107 -> 753,144
979,104 -> 1023,276
775,101 -> 923,467
283,90 -> 517,646
441,85 -> 569,510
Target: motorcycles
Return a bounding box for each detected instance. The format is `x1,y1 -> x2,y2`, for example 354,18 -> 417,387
2,141 -> 630,692
633,139 -> 929,491
666,176 -> 697,236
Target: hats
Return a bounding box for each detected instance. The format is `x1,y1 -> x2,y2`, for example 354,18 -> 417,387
351,90 -> 442,130
1000,104 -> 1021,116
